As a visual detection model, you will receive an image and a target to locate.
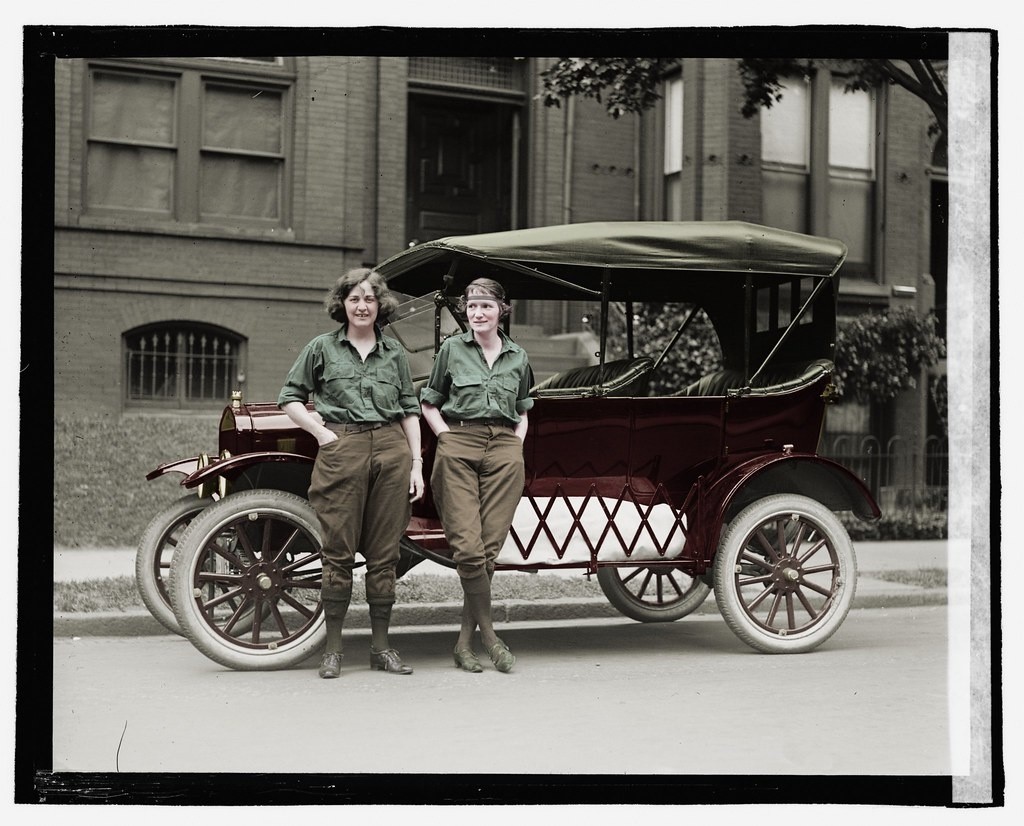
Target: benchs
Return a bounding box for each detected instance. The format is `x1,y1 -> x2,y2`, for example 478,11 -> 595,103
664,355 -> 836,396
522,353 -> 660,397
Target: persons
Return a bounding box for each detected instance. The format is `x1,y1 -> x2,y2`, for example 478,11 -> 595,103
419,278 -> 535,672
278,268 -> 425,678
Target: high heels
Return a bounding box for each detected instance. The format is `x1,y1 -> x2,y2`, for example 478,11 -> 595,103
454,644 -> 483,672
487,637 -> 516,672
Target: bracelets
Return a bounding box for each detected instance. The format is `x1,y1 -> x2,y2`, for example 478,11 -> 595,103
412,457 -> 423,464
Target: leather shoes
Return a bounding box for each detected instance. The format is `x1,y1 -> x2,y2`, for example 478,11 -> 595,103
319,651 -> 344,678
370,646 -> 413,674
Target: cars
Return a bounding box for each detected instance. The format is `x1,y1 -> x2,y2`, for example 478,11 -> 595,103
136,219 -> 884,671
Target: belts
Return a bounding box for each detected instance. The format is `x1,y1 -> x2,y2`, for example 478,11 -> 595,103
326,422 -> 390,433
450,418 -> 513,427
